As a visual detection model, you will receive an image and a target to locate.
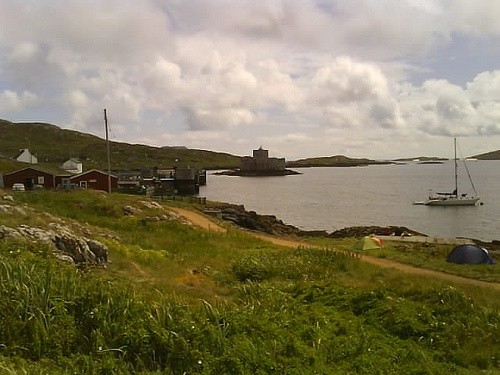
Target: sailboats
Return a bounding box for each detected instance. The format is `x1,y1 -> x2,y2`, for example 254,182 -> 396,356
424,137 -> 480,206
